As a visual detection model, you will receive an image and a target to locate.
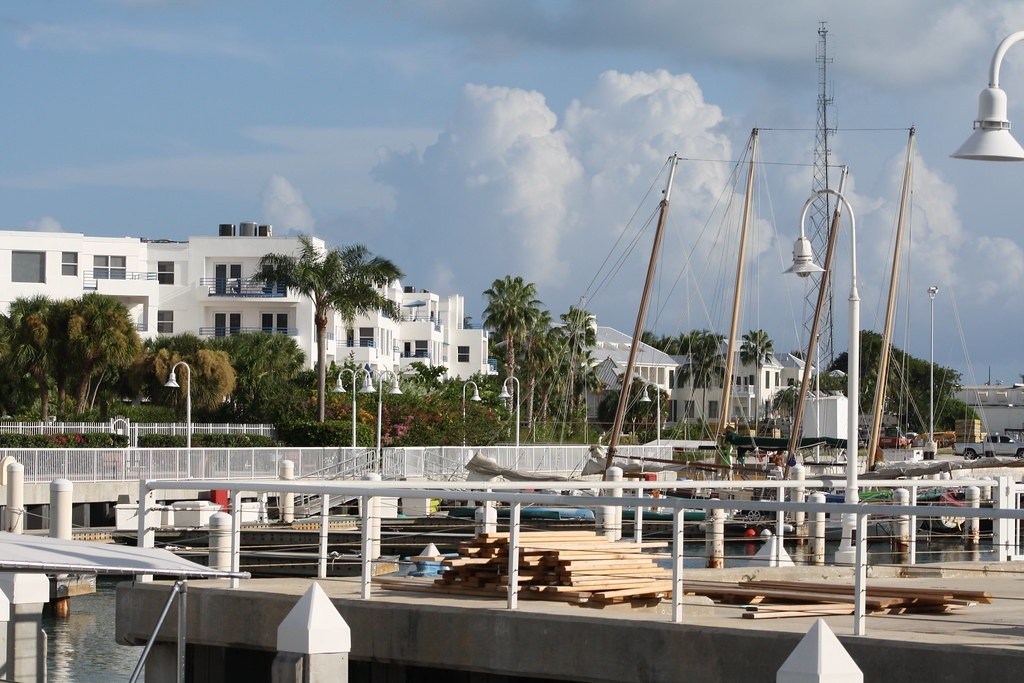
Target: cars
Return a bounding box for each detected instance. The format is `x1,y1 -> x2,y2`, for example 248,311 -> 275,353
904,431 -> 917,445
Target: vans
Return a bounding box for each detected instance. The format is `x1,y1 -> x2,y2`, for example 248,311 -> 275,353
877,424 -> 906,449
912,431 -> 957,449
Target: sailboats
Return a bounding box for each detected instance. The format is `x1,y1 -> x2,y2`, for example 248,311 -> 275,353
433,119 -> 1024,556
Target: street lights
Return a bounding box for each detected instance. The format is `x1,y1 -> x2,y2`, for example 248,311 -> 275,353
639,382 -> 660,461
357,370 -> 403,478
333,367 -> 377,477
460,380 -> 482,472
164,362 -> 191,478
927,285 -> 938,455
498,376 -> 519,471
780,188 -> 860,524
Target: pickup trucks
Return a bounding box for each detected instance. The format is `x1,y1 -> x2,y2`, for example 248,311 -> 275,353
950,435 -> 1024,461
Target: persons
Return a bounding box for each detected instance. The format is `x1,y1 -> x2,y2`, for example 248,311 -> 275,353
771,449 -> 785,480
